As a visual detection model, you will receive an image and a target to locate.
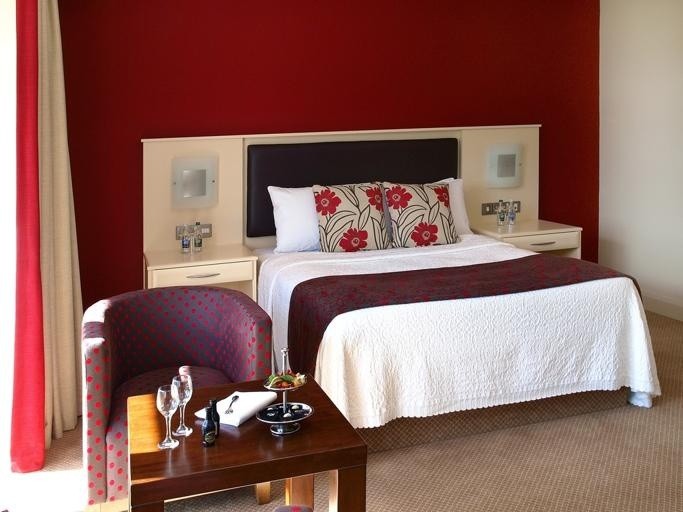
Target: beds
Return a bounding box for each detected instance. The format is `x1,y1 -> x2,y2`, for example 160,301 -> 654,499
254,236 -> 661,452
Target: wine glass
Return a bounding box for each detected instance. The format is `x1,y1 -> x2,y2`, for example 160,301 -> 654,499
171,375 -> 194,437
156,384 -> 180,450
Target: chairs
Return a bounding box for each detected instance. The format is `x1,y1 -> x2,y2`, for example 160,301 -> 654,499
81,285 -> 273,511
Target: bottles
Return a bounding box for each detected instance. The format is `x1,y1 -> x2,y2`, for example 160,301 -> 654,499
507,200 -> 517,225
193,221 -> 204,253
200,407 -> 216,448
208,399 -> 220,437
497,199 -> 506,226
182,224 -> 192,253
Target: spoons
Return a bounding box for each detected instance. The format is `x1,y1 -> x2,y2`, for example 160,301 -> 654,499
225,395 -> 239,414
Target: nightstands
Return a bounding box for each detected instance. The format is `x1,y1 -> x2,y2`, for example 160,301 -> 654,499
141,243 -> 258,303
469,219 -> 583,260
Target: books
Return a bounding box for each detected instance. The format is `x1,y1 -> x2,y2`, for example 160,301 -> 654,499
194,391 -> 277,427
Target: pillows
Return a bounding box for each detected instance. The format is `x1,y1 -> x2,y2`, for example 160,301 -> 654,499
266,178 -> 474,253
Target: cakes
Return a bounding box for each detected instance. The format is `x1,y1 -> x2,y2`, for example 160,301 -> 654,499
259,403 -> 309,421
